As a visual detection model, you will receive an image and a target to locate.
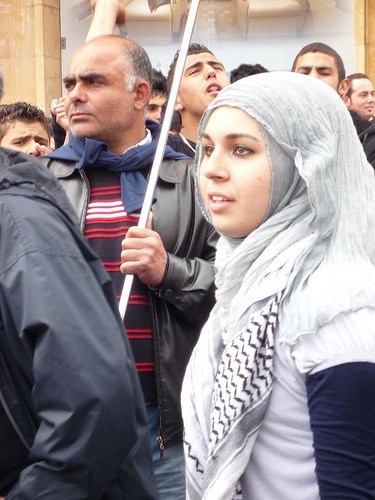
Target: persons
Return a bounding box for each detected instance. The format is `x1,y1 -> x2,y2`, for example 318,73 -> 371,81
0,0 -> 375,500
